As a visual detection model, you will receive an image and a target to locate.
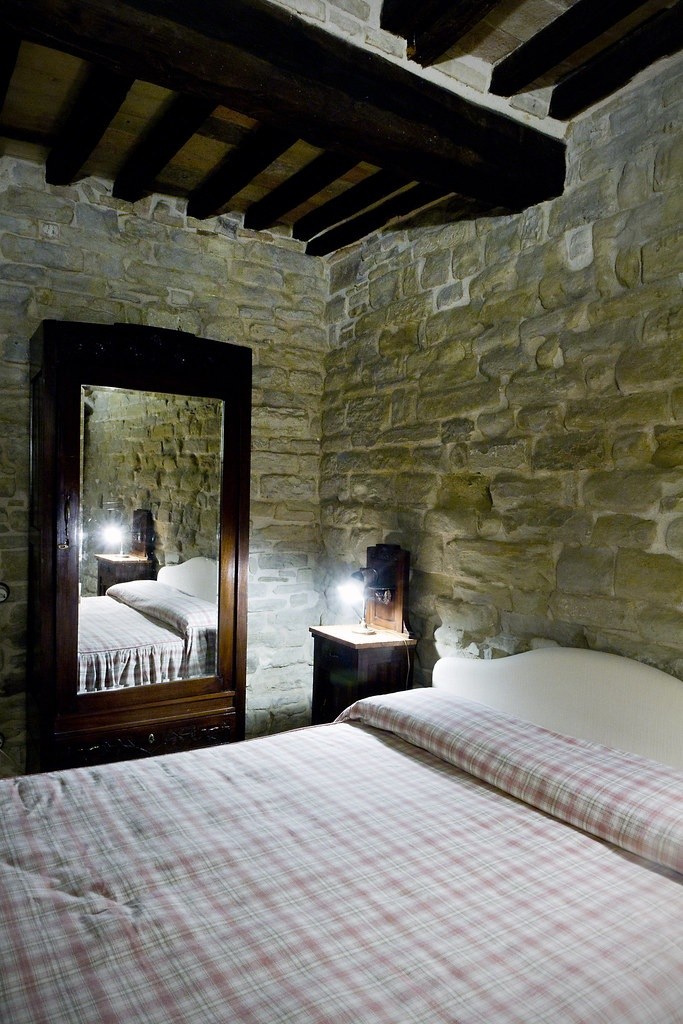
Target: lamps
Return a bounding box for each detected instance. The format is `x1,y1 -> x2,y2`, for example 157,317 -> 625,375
351,567 -> 391,591
106,522 -> 132,556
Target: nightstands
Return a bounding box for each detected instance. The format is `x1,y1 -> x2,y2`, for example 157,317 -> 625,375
94,510 -> 153,596
310,544 -> 417,725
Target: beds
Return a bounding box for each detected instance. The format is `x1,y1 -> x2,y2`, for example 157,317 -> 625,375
0,648 -> 683,1024
77,557 -> 217,695
